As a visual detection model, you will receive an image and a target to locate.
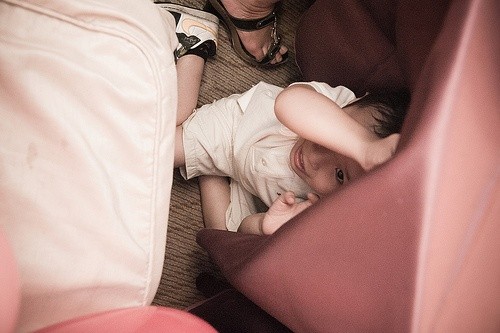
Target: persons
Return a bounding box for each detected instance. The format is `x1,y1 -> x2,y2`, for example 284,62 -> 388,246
157,2 -> 408,239
207,0 -> 289,68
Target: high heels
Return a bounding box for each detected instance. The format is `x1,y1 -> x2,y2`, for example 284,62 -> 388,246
203,0 -> 290,70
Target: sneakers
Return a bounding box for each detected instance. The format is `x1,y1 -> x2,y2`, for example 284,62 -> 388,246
153,1 -> 219,59
